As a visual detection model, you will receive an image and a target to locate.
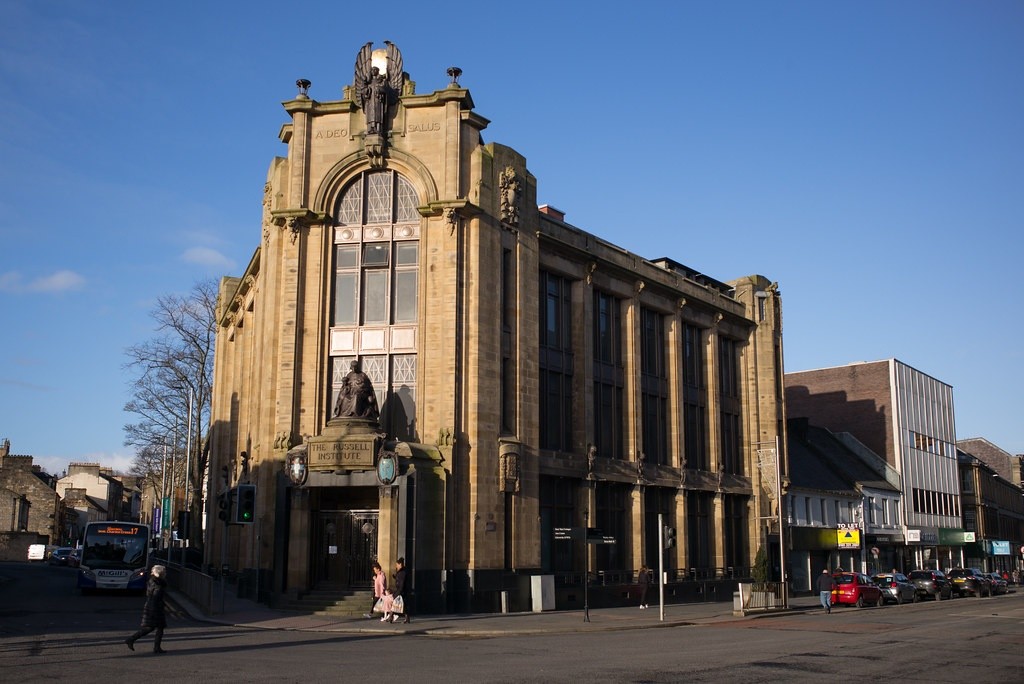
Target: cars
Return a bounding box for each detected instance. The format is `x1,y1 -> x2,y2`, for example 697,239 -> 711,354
68,549 -> 82,568
870,573 -> 918,605
830,572 -> 885,608
51,548 -> 72,565
984,572 -> 1008,596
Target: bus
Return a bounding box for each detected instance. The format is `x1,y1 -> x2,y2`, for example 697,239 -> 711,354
76,519 -> 151,593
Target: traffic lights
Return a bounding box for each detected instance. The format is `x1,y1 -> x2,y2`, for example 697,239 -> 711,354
236,484 -> 256,525
664,526 -> 676,549
218,499 -> 230,521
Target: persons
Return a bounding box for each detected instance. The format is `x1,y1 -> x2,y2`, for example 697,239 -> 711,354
816,570 -> 837,615
638,455 -> 646,478
334,360 -> 380,419
92,543 -> 105,560
361,66 -> 387,134
638,565 -> 650,609
681,460 -> 686,484
241,451 -> 248,477
126,565 -> 169,654
717,466 -> 725,488
892,569 -> 896,573
363,557 -> 410,624
150,549 -> 160,558
996,570 -> 1024,588
588,446 -> 597,472
222,466 -> 229,486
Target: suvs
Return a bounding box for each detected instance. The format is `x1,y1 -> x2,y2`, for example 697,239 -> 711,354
906,570 -> 953,601
947,567 -> 991,598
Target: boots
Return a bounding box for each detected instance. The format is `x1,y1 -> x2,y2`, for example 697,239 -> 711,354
384,613 -> 394,623
401,614 -> 410,625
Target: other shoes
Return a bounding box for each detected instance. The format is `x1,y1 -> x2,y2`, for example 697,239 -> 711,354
645,604 -> 648,609
639,605 -> 645,609
125,638 -> 135,652
363,613 -> 371,619
824,606 -> 829,614
828,612 -> 831,614
380,618 -> 384,622
153,647 -> 167,654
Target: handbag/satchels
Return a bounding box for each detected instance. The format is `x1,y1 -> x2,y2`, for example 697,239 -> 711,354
373,596 -> 390,612
391,595 -> 404,614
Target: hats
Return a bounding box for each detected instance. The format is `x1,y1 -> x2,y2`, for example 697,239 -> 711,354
823,569 -> 828,573
